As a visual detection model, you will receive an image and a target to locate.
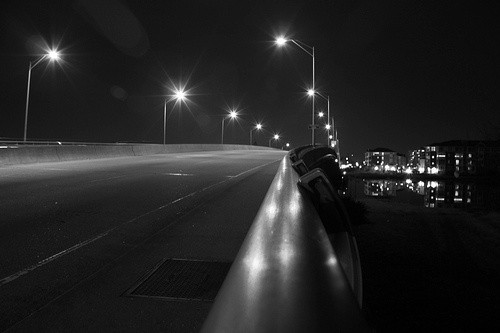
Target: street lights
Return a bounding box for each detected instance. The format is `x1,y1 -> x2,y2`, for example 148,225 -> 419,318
23,52 -> 60,144
222,110 -> 236,144
325,124 -> 340,167
163,92 -> 184,144
277,38 -> 316,145
282,143 -> 289,150
250,124 -> 261,144
319,112 -> 334,151
307,89 -> 329,146
269,135 -> 278,148
